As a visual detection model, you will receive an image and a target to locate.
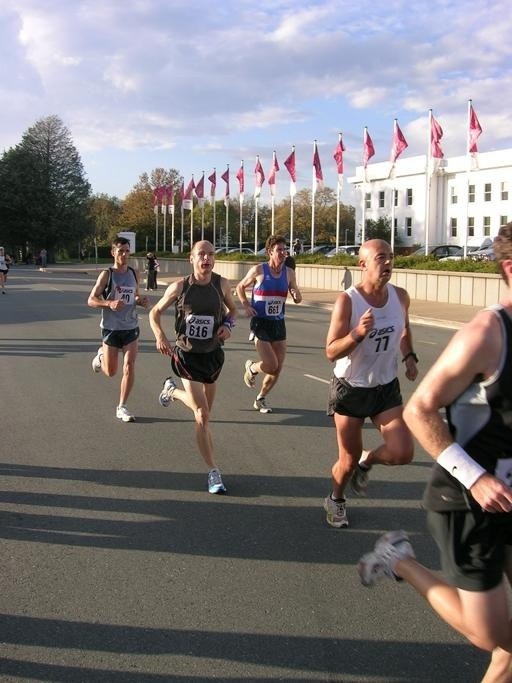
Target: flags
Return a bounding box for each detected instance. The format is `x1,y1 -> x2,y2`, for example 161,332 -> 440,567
430,115 -> 443,159
467,107 -> 482,156
153,129 -> 375,213
393,123 -> 408,162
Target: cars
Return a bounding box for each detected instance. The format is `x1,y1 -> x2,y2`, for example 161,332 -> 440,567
212,236 -> 499,262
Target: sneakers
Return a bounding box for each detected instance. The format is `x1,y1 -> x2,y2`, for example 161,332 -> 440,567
207,466 -> 227,494
252,396 -> 273,415
243,359 -> 258,389
116,405 -> 136,423
323,489 -> 350,530
355,530 -> 417,592
91,345 -> 105,374
1,280 -> 8,294
158,376 -> 177,408
144,286 -> 158,292
350,448 -> 373,499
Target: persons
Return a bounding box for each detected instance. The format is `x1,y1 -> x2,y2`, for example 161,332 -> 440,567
153,255 -> 160,289
149,239 -> 235,496
11,247 -> 48,268
285,251 -> 296,270
324,238 -> 418,528
88,237 -> 149,423
235,235 -> 302,411
144,252 -> 155,292
292,239 -> 301,255
80,248 -> 86,263
357,226 -> 511,681
0,246 -> 12,294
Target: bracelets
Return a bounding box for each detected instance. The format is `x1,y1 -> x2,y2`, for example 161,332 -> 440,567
436,442 -> 488,491
223,316 -> 236,331
401,353 -> 418,363
350,328 -> 364,343
